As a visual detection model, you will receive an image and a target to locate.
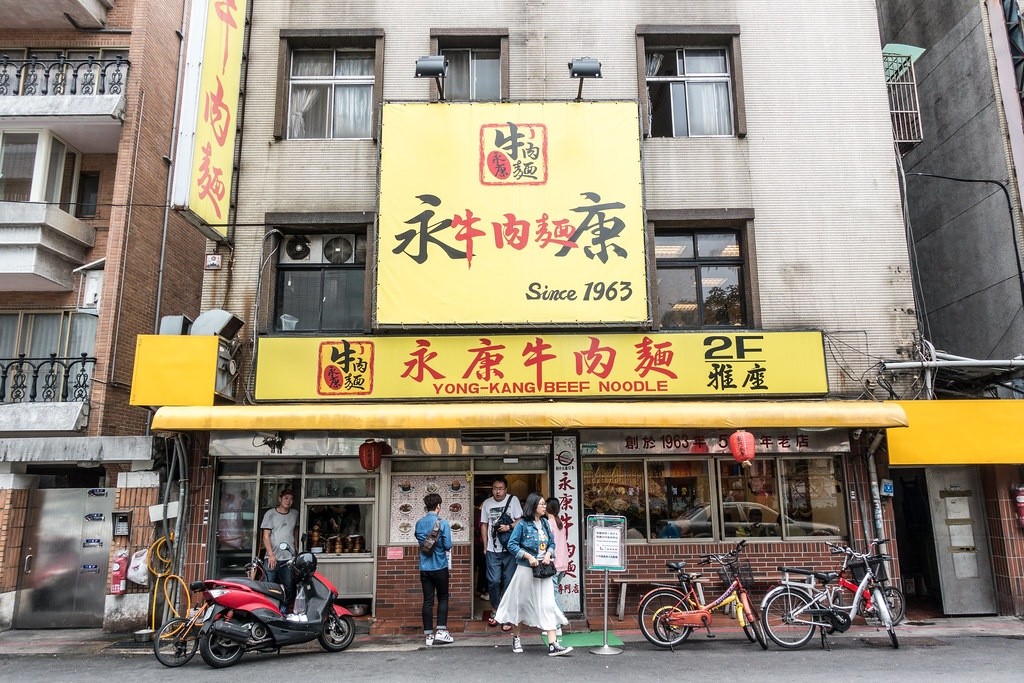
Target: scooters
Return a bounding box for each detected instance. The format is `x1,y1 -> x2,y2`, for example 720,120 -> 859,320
190,532 -> 355,668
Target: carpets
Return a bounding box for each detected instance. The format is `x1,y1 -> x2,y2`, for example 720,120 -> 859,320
541,629 -> 624,649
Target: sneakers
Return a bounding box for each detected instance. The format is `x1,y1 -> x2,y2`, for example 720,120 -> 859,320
548,639 -> 573,656
512,636 -> 523,653
426,634 -> 435,646
435,630 -> 453,642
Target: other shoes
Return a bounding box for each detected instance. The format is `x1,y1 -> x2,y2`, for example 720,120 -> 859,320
542,628 -> 563,637
481,593 -> 490,601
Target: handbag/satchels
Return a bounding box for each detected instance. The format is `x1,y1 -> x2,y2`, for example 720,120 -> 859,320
293,587 -> 307,615
533,561 -> 557,578
494,514 -> 515,552
127,549 -> 149,586
420,534 -> 438,557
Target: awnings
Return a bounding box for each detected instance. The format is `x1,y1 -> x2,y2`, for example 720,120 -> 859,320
149,404 -> 908,433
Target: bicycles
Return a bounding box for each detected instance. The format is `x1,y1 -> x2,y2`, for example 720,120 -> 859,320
153,581 -> 206,667
632,539 -> 768,654
824,542 -> 907,628
760,538 -> 900,651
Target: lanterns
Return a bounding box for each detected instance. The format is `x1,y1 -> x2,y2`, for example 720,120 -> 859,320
359,438 -> 380,475
729,429 -> 755,468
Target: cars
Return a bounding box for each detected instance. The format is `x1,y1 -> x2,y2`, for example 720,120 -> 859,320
655,501 -> 841,538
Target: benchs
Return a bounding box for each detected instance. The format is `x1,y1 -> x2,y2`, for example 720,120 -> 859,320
722,573 -> 811,619
612,578 -> 709,621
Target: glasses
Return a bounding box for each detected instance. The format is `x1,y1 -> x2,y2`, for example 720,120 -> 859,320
538,501 -> 548,507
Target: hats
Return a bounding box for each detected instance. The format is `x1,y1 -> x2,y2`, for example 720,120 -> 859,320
280,489 -> 294,497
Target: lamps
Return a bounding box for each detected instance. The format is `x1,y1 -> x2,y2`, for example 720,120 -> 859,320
568,54 -> 602,101
414,55 -> 449,103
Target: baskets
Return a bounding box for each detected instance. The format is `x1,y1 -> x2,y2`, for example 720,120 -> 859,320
716,559 -> 754,590
849,555 -> 888,586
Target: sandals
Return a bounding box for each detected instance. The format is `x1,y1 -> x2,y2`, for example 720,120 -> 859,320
501,622 -> 513,632
488,611 -> 498,627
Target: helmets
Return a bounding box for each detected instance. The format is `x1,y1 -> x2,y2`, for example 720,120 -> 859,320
292,552 -> 318,582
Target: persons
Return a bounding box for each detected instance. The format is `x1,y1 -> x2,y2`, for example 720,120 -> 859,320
672,480 -> 774,510
473,476 -> 568,637
493,492 -> 573,657
625,508 -> 807,539
260,488 -> 299,616
310,487 -> 361,538
414,494 -> 454,646
219,490 -> 255,536
